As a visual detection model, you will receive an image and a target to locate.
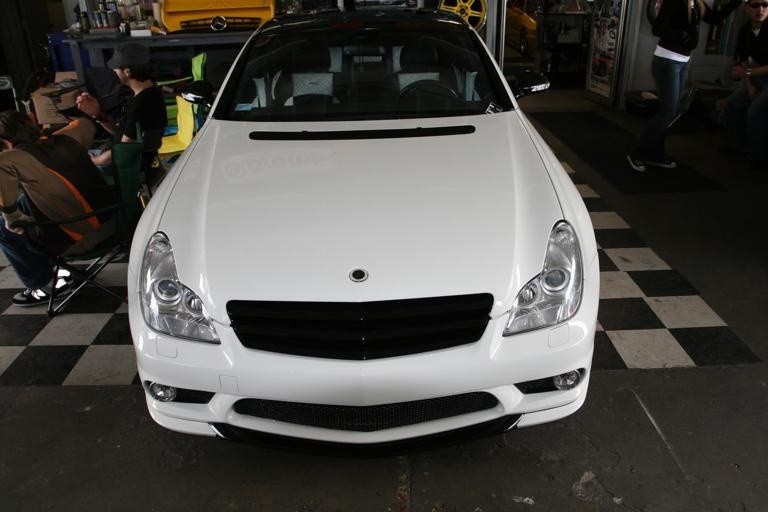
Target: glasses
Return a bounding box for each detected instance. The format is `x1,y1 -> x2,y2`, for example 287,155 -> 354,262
747,2 -> 768,9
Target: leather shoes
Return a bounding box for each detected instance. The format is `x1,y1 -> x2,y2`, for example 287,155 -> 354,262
749,159 -> 765,170
718,145 -> 748,156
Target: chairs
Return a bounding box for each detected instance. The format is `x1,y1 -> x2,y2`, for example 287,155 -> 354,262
271,42 -> 457,105
11,141 -> 143,317
154,53 -> 206,122
156,94 -> 195,154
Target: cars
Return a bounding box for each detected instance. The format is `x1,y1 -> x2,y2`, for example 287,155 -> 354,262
125,5 -> 603,449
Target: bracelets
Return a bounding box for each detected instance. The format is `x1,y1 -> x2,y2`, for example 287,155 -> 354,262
745,68 -> 752,79
94,109 -> 105,124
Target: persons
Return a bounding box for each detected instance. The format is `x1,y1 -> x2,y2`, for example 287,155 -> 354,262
625,1 -> 749,173
717,1 -> 768,169
0,111 -> 115,307
74,42 -> 168,186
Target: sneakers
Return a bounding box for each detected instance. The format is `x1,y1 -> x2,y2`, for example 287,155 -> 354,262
11,277 -> 73,307
645,155 -> 677,169
626,147 -> 647,173
53,265 -> 76,287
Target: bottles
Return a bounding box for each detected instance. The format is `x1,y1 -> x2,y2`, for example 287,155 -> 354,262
86,0 -> 121,29
128,16 -> 136,29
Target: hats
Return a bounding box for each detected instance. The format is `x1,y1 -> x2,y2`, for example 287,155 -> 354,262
106,42 -> 152,68
